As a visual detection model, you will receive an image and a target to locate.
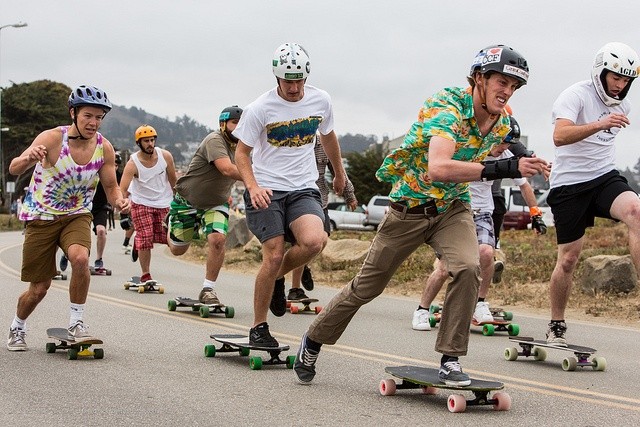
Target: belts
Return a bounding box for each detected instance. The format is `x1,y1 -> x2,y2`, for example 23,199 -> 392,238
390,201 -> 437,216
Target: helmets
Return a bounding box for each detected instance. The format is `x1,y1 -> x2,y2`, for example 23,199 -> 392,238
591,42 -> 640,107
135,125 -> 157,142
219,105 -> 243,121
273,43 -> 310,81
113,150 -> 122,162
470,44 -> 529,90
503,118 -> 520,144
67,85 -> 112,110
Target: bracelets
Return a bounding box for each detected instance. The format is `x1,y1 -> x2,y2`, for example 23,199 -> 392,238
529,205 -> 542,216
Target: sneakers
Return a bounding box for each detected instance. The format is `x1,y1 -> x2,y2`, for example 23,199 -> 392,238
270,277 -> 287,317
412,309 -> 431,331
141,273 -> 152,282
438,358 -> 471,386
301,265 -> 314,291
473,302 -> 493,323
546,322 -> 567,347
199,287 -> 219,304
67,320 -> 94,342
288,288 -> 309,299
132,240 -> 138,262
95,260 -> 103,269
7,326 -> 28,351
60,255 -> 68,271
122,244 -> 131,251
249,322 -> 279,347
493,261 -> 504,284
293,333 -> 319,384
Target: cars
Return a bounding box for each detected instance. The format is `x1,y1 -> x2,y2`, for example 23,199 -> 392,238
328,201 -> 375,232
527,189 -> 556,229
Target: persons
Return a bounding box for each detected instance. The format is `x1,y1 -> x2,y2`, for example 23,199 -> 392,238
491,139 -> 552,283
5,84 -> 132,353
292,43 -> 549,388
288,133 -> 358,299
230,42 -> 347,347
544,41 -> 640,347
108,205 -> 116,230
161,105 -> 243,304
119,123 -> 178,282
411,115 -> 547,331
58,152 -> 122,271
122,211 -> 135,251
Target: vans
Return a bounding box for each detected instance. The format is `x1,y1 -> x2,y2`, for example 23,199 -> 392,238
501,187 -> 547,228
362,194 -> 393,226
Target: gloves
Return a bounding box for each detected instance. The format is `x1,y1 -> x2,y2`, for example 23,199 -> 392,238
532,214 -> 547,234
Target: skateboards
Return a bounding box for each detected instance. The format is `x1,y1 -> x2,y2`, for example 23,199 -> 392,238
430,303 -> 513,320
429,313 -> 519,336
204,334 -> 296,370
124,276 -> 164,294
168,297 -> 234,318
52,271 -> 66,280
285,296 -> 322,314
46,328 -> 104,360
379,365 -> 511,412
504,336 -> 606,371
89,266 -> 111,275
123,244 -> 132,254
